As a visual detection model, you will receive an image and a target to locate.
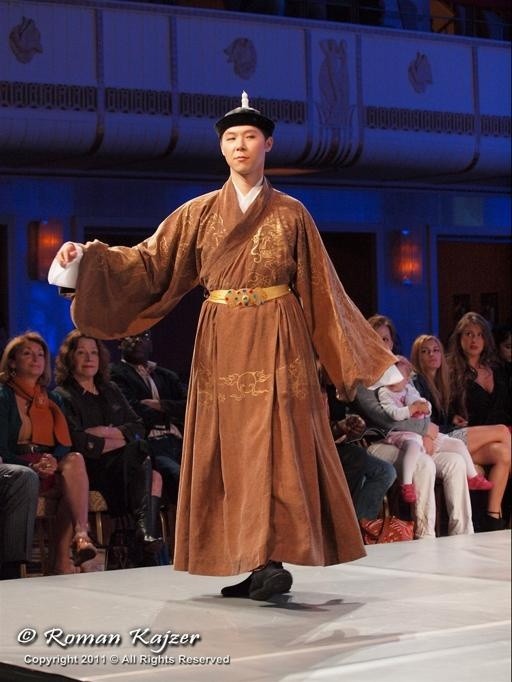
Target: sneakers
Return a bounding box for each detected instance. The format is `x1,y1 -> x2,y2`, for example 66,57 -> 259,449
466,471 -> 496,492
484,506 -> 507,531
68,521 -> 99,568
220,560 -> 294,602
399,483 -> 420,505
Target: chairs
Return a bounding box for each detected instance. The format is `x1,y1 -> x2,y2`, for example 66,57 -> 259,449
157,502 -> 176,566
20,483 -> 63,576
339,463 -> 392,542
88,489 -> 136,570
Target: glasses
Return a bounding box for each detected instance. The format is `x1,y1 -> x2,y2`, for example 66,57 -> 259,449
135,333 -> 152,342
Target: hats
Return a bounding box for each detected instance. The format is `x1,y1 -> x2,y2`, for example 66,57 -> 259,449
213,88 -> 277,141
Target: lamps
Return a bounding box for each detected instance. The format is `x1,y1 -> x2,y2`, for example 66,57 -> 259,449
388,224 -> 423,288
26,213 -> 66,286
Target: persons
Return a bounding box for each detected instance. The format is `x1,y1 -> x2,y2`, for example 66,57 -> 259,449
1,460 -> 40,581
57,106 -> 392,602
109,326 -> 187,504
378,353 -> 495,502
315,358 -> 398,524
495,322 -> 512,363
0,330 -> 98,575
406,335 -> 511,529
447,311 -> 512,427
354,313 -> 479,541
50,327 -> 164,573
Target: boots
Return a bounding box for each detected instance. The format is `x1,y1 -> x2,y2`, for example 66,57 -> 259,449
141,494 -> 164,569
129,456 -> 165,556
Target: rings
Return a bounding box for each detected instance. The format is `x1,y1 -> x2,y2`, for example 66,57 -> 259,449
46,462 -> 52,469
